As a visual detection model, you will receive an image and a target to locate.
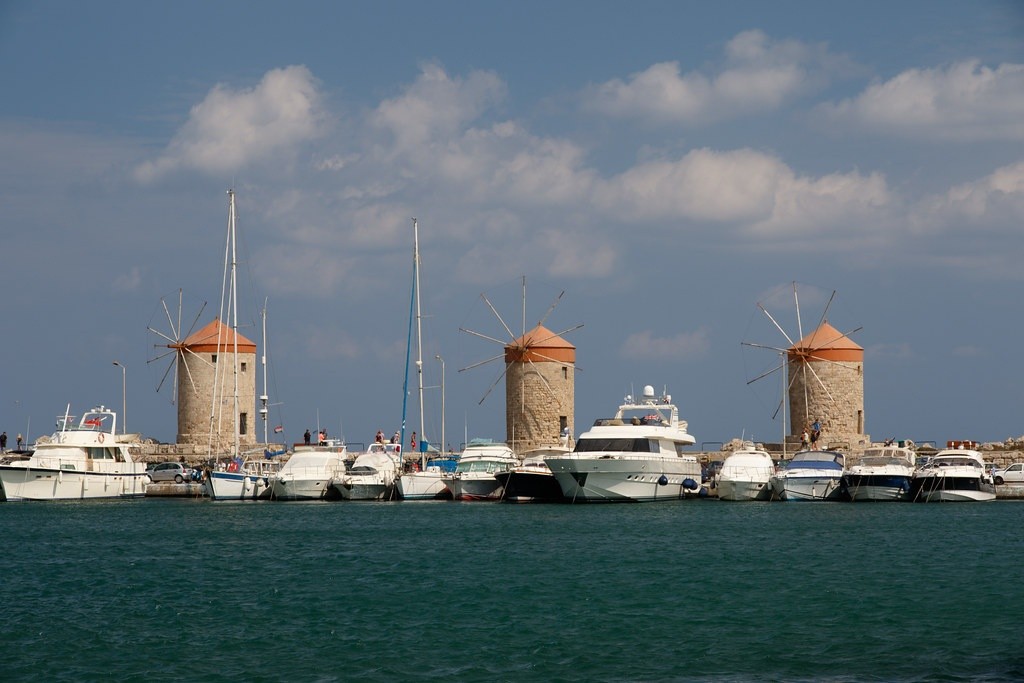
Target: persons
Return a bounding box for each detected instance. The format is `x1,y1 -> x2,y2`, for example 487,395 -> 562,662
392,431 -> 400,452
0,431 -> 7,451
16,433 -> 23,451
319,432 -> 326,446
799,427 -> 820,450
810,418 -> 822,430
304,429 -> 310,445
560,423 -> 571,448
375,430 -> 384,451
411,431 -> 417,451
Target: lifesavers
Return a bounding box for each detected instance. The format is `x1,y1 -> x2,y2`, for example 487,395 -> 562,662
412,463 -> 418,472
98,432 -> 104,443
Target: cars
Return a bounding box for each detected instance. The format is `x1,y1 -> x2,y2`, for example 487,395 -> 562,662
984,462 -> 1024,484
147,462 -> 192,483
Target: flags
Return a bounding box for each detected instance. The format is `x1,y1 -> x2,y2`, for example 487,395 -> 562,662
274,425 -> 282,432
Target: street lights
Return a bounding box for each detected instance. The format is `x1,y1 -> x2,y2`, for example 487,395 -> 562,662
435,354 -> 445,461
114,360 -> 126,434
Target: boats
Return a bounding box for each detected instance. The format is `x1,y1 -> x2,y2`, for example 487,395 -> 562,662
270,445 -> 344,500
0,404 -> 149,501
843,446 -> 914,502
333,442 -> 401,501
714,450 -> 775,502
440,446 -> 515,501
914,450 -> 995,501
771,451 -> 843,501
493,455 -> 562,502
541,384 -> 702,502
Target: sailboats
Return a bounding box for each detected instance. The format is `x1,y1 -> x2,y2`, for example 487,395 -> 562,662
394,217 -> 450,500
204,191 -> 267,499
